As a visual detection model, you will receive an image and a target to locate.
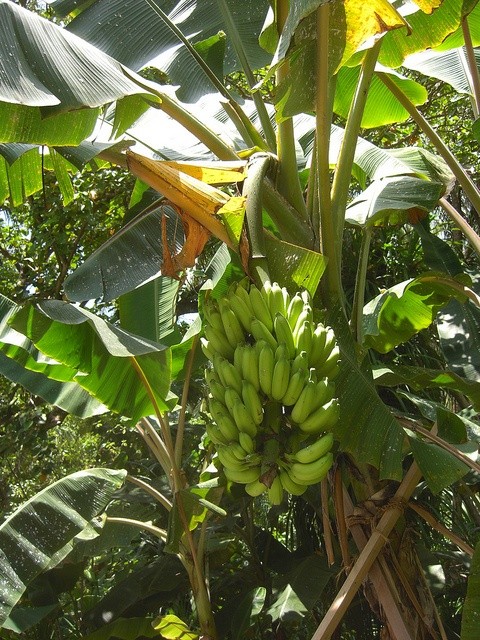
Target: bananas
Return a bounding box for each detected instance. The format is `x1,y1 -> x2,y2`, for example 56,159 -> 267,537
201,279 -> 343,505
